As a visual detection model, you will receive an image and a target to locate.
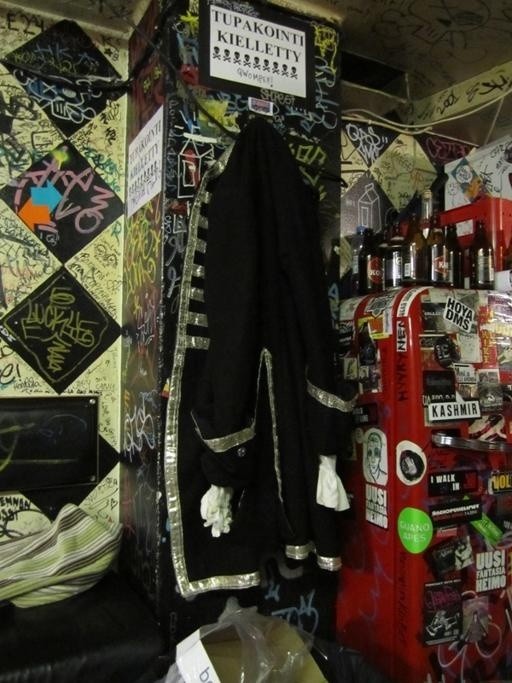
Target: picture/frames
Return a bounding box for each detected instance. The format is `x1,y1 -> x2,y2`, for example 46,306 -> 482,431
199,0 -> 316,111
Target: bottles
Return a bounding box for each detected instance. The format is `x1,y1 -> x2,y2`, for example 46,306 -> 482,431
350,186 -> 496,293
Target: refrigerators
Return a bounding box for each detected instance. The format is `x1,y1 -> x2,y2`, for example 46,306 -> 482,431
328,283 -> 512,683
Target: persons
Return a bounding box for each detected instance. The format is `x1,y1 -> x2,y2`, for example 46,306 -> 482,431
359,368 -> 380,394
460,611 -> 487,642
364,431 -> 389,485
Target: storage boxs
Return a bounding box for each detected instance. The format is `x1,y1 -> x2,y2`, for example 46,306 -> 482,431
176,616 -> 328,683
